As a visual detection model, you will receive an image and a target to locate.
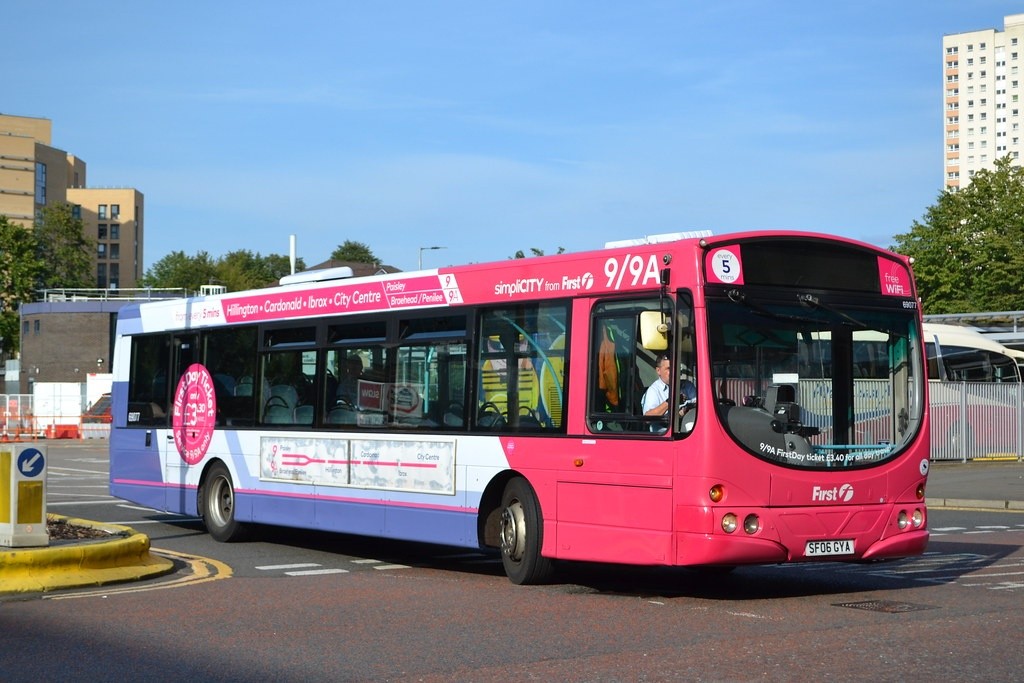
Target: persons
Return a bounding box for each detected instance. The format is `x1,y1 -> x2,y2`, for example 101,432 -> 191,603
336,355 -> 363,404
641,353 -> 696,433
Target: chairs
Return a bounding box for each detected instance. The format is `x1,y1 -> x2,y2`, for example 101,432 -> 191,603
135,371 -> 544,430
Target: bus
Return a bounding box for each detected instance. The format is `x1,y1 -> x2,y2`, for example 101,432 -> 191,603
105,222 -> 934,585
793,323 -> 1024,458
